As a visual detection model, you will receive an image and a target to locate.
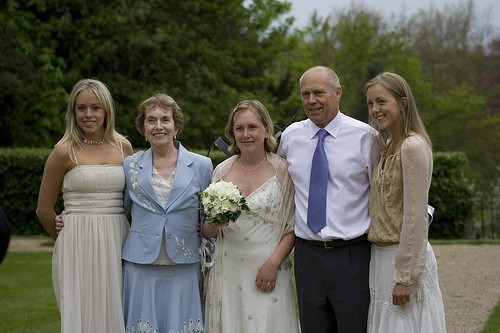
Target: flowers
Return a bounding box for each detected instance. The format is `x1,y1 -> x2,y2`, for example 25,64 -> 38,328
196,178 -> 251,227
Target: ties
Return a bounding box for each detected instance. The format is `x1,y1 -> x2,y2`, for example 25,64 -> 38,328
306,129 -> 330,235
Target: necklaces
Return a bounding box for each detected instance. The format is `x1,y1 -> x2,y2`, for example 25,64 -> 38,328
238,157 -> 266,172
82,136 -> 108,146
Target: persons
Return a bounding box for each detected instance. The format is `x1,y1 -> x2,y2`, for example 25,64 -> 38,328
199,100 -> 302,333
38,78 -> 139,333
362,71 -> 448,333
56,90 -> 213,333
273,65 -> 433,333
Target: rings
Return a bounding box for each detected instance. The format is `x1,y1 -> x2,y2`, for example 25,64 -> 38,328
266,282 -> 271,286
